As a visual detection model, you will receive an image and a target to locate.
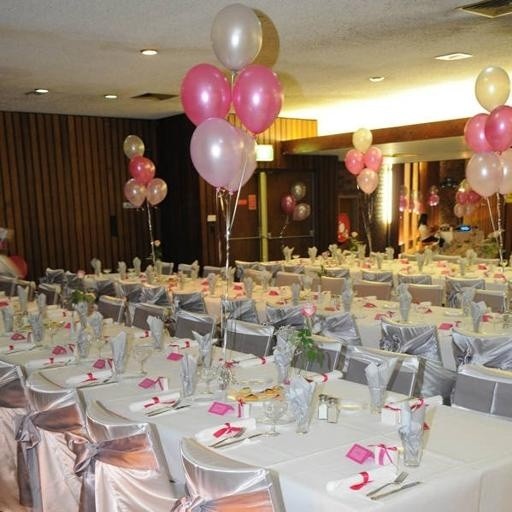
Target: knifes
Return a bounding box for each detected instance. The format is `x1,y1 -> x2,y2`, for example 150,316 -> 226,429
146,403 -> 192,417
213,432 -> 263,449
370,479 -> 421,499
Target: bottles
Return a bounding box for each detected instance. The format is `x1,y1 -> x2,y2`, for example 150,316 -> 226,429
318,393 -> 339,424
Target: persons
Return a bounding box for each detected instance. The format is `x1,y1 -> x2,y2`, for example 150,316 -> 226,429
416,213 -> 445,250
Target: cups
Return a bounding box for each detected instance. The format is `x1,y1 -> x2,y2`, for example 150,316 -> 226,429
294,402 -> 314,435
397,428 -> 424,468
3,299 -> 292,398
92,257 -> 482,332
368,385 -> 387,417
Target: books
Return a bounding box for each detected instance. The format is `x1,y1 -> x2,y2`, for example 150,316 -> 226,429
463,66 -> 512,198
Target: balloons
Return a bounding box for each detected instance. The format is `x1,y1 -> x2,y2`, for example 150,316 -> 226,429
399,178 -> 482,218
356,168 -> 379,195
289,181 -> 306,201
179,64 -> 232,128
122,135 -> 168,210
280,194 -> 296,215
364,147 -> 382,171
292,203 -> 311,221
503,195 -> 512,205
223,124 -> 258,193
234,66 -> 284,134
210,3 -> 263,72
352,127 -> 372,154
189,116 -> 244,188
345,148 -> 364,176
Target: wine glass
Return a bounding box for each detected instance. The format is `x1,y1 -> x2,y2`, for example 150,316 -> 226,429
261,401 -> 288,437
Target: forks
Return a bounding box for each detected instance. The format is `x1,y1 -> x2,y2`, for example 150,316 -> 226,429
363,470 -> 410,497
146,399 -> 182,414
208,428 -> 245,451
